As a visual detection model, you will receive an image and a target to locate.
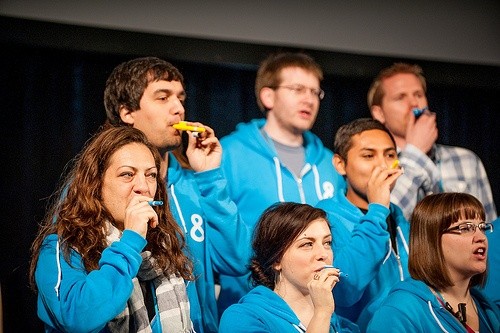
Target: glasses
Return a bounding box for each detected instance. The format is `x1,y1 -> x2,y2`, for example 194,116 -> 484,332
440,222 -> 494,235
270,84 -> 324,99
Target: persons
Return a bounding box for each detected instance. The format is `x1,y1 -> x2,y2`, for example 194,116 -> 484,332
215,52 -> 347,318
315,120 -> 425,333
104,55 -> 253,332
219,201 -> 362,332
367,192 -> 500,333
367,62 -> 500,224
29,126 -> 197,333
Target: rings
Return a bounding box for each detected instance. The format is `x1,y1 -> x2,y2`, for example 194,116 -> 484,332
313,272 -> 322,281
